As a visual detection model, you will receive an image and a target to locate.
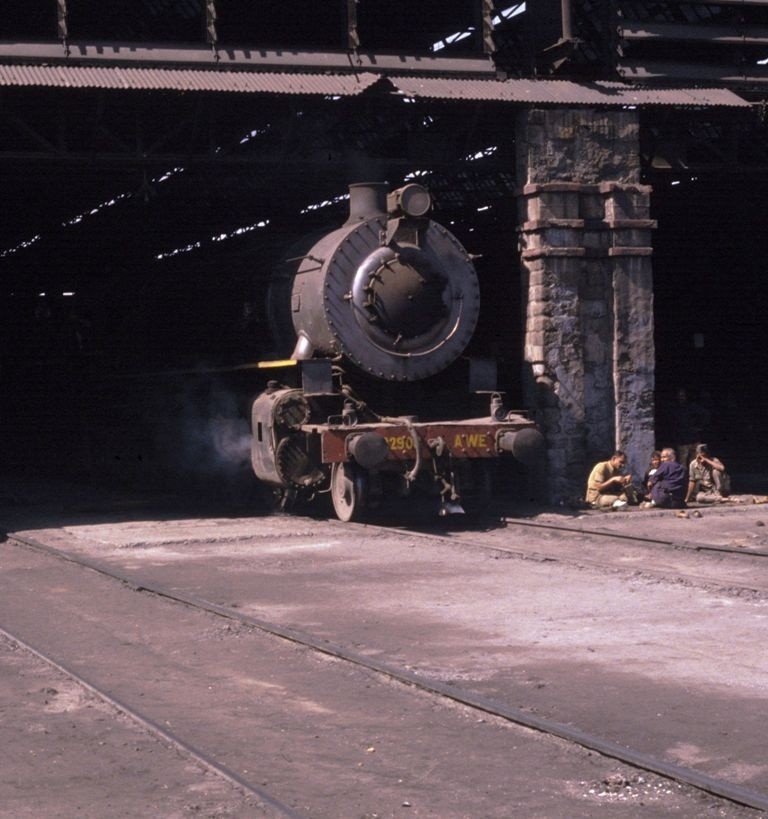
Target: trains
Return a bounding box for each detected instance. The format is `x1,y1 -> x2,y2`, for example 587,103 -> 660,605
101,177 -> 549,523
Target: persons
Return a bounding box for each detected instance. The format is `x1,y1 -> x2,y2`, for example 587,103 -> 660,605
639,444 -> 732,510
586,450 -> 638,507
